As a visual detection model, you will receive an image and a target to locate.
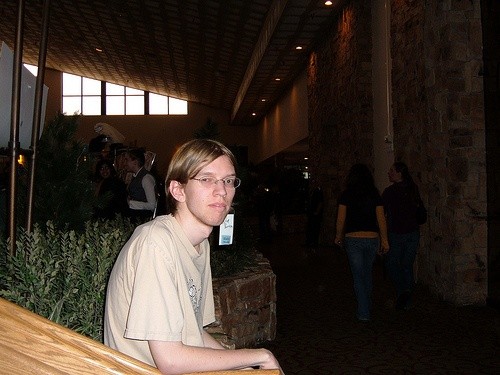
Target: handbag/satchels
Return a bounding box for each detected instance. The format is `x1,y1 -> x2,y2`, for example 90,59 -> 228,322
416,186 -> 427,225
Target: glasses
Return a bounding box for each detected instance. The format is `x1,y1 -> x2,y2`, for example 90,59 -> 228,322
99,167 -> 110,170
125,158 -> 134,162
193,175 -> 241,188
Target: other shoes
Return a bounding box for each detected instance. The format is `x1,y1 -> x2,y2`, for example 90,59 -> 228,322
393,293 -> 412,311
359,318 -> 369,320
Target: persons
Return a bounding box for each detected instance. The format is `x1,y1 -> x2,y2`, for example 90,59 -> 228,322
87,134 -> 168,223
381,161 -> 427,309
231,160 -> 325,257
104,138 -> 285,375
334,162 -> 390,321
